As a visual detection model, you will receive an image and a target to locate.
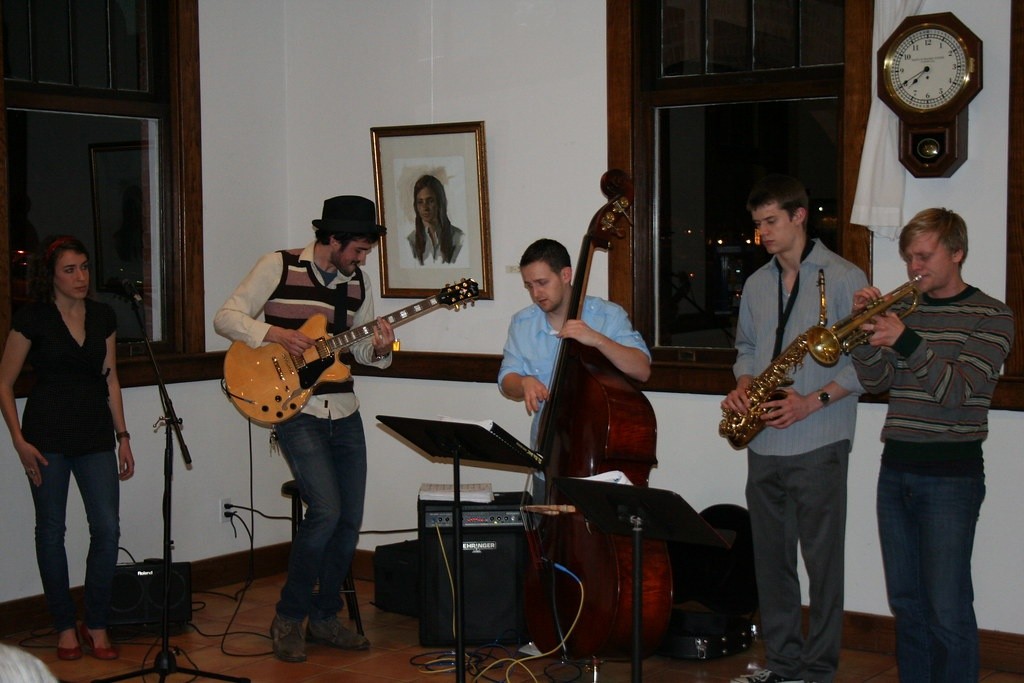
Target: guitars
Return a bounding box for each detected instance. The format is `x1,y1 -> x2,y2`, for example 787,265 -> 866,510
223,277 -> 480,430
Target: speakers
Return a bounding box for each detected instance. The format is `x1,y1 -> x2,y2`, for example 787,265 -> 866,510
417,491 -> 534,646
106,557 -> 193,641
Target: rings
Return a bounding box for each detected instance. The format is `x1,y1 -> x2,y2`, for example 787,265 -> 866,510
28,471 -> 36,477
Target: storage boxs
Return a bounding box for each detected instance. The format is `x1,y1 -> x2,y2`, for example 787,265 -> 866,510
373,538 -> 418,617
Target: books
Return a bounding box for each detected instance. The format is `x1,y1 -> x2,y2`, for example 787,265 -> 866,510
438,414 -> 543,466
569,471 -> 633,485
418,483 -> 495,503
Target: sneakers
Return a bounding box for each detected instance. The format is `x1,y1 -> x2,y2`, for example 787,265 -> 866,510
305,616 -> 369,649
730,669 -> 789,683
270,614 -> 306,661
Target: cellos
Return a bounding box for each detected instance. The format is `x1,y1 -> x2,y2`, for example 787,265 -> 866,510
519,167 -> 673,683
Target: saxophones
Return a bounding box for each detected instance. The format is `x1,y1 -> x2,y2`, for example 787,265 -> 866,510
718,268 -> 828,451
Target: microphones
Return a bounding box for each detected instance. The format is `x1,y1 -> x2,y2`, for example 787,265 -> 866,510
116,273 -> 143,302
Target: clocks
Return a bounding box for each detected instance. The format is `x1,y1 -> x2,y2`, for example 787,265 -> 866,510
876,12 -> 986,178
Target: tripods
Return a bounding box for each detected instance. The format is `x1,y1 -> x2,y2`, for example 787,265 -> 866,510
90,300 -> 251,683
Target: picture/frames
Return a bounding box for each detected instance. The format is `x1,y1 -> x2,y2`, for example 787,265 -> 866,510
366,119 -> 496,300
86,141 -> 145,297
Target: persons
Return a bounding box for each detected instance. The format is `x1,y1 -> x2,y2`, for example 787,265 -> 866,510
853,207 -> 1013,683
497,238 -> 652,655
721,175 -> 867,683
0,235 -> 135,660
214,196 -> 394,662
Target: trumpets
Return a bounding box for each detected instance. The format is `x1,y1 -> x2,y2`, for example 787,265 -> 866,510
805,274 -> 923,365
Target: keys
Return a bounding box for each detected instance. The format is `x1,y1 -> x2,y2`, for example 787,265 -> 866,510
269,429 -> 282,457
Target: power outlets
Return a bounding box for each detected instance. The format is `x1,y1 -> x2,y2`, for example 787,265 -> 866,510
219,496 -> 232,522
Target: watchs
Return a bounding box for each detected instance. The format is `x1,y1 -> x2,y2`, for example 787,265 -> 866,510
816,388 -> 830,408
374,353 -> 392,361
115,430 -> 132,439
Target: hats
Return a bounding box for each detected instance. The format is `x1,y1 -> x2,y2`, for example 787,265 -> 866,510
312,196 -> 387,235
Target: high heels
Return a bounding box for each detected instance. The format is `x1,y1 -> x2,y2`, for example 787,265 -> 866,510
57,626 -> 81,660
80,622 -> 118,659
